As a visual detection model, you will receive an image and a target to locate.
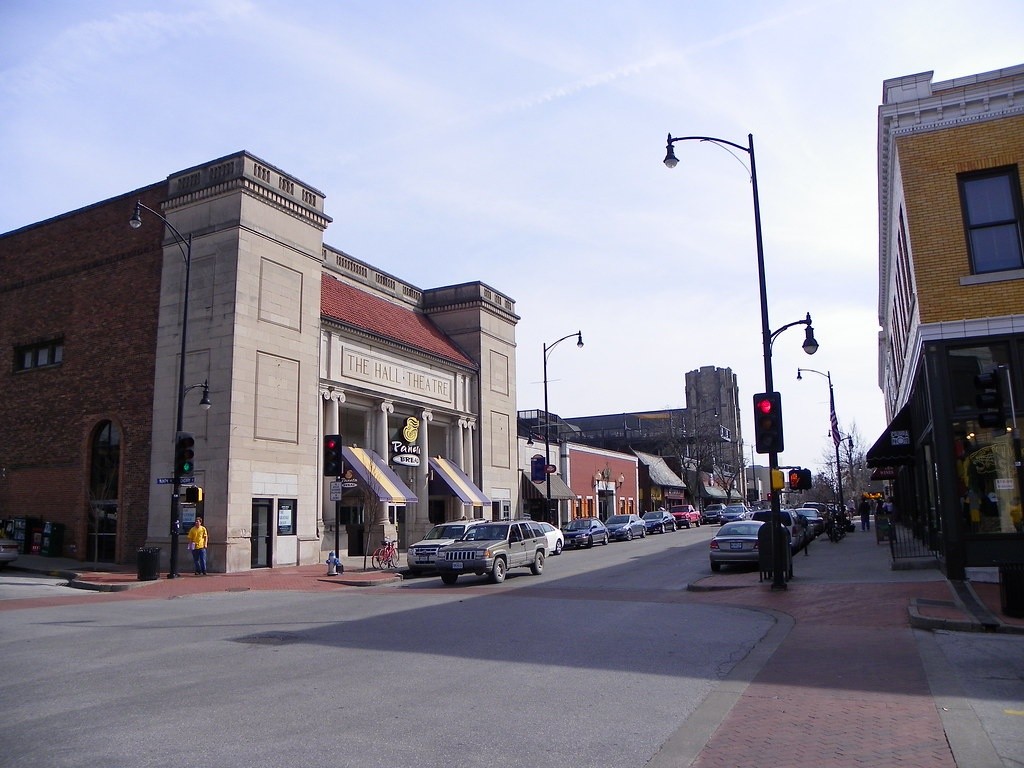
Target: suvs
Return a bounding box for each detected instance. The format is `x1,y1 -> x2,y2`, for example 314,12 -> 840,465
405,515 -> 550,584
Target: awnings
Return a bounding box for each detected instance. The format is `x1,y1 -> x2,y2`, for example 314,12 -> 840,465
866,402 -> 915,481
522,471 -> 577,501
701,487 -> 743,499
342,446 -> 418,503
428,457 -> 491,506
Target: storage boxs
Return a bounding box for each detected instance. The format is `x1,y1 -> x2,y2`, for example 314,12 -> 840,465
4,516 -> 65,556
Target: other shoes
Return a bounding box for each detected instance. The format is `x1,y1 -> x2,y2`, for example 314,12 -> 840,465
195,571 -> 201,575
859,529 -> 865,532
203,570 -> 206,575
867,527 -> 870,530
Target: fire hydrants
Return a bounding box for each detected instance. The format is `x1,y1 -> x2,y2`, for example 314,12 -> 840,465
326,550 -> 340,575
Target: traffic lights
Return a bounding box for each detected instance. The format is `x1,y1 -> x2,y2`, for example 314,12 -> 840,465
174,431 -> 195,477
788,469 -> 812,489
323,435 -> 342,476
771,469 -> 784,491
753,392 -> 785,454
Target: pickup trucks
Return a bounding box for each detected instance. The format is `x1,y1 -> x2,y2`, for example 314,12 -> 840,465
669,504 -> 702,529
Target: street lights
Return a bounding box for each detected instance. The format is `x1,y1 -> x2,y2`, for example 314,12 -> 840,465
128,196 -> 212,578
797,367 -> 854,513
692,408 -> 720,526
543,332 -> 584,524
664,132 -> 820,592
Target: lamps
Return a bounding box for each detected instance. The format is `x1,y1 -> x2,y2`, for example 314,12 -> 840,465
594,468 -> 603,487
616,472 -> 625,490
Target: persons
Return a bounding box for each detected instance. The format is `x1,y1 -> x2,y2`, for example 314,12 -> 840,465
875,496 -> 893,525
187,517 -> 208,575
658,505 -> 665,511
857,497 -> 870,531
847,496 -> 855,521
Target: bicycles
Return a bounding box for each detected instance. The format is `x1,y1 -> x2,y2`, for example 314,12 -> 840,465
371,539 -> 402,569
826,516 -> 843,542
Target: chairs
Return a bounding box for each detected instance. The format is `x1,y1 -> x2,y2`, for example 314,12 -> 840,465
496,529 -> 507,538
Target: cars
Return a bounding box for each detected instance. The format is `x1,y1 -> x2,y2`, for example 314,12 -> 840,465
640,499 -> 831,572
559,516 -> 610,549
604,514 -> 648,542
536,522 -> 565,555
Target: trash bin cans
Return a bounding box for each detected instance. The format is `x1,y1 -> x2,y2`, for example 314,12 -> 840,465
137,546 -> 162,581
993,559 -> 1024,618
757,521 -> 792,582
